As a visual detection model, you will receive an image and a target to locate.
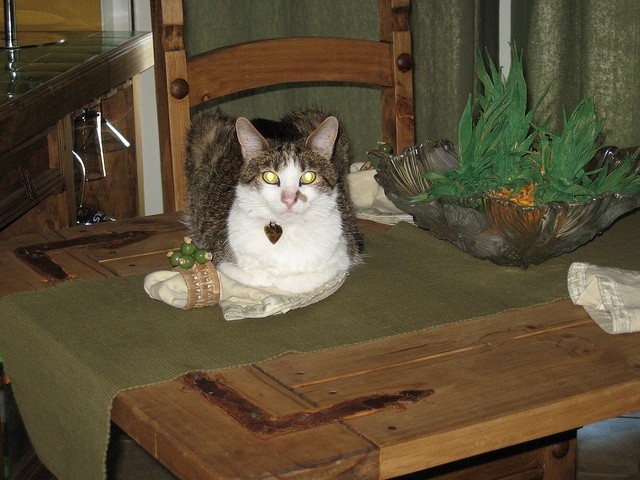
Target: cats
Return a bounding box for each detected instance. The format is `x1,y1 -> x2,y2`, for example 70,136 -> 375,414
177,104 -> 373,293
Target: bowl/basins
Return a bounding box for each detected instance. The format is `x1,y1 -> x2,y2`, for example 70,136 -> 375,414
367,137 -> 639,270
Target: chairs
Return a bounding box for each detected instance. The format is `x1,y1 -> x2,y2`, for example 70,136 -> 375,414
146,1 -> 427,212
